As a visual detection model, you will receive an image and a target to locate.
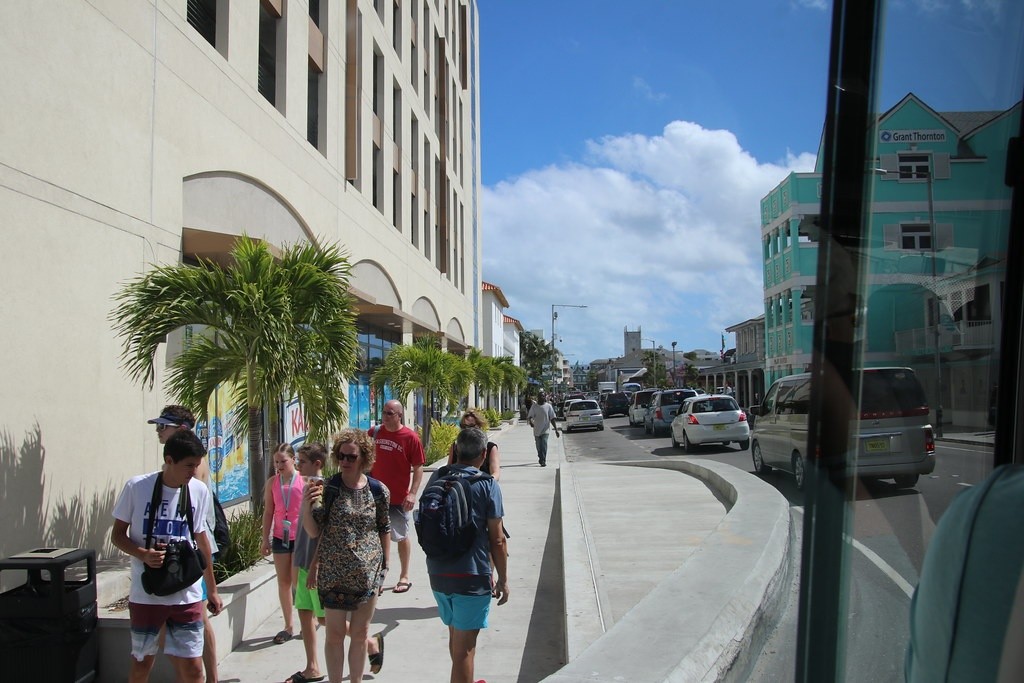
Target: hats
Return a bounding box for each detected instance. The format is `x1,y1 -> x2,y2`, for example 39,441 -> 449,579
148,414 -> 193,430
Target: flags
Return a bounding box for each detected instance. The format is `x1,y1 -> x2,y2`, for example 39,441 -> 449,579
722,335 -> 725,349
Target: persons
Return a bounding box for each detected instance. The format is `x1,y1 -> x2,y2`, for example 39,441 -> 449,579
546,391 -> 565,404
259,442 -> 308,645
527,391 -> 560,467
446,409 -> 501,598
147,404 -> 210,683
426,428 -> 509,683
725,383 -> 732,393
302,430 -> 392,683
285,440 -> 384,683
367,398 -> 426,597
112,428 -> 223,683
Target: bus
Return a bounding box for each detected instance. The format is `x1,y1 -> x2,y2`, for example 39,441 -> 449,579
622,383 -> 641,392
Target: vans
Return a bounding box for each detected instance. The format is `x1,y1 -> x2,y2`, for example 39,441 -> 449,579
749,366 -> 934,490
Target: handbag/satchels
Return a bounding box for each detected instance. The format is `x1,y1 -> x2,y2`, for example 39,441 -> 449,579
141,471 -> 207,597
213,492 -> 231,559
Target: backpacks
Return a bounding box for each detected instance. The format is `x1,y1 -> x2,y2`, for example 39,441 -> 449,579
414,467 -> 493,558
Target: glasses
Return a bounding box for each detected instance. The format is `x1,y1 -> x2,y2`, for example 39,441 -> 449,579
336,452 -> 361,463
382,410 -> 400,415
157,421 -> 180,431
461,423 -> 480,429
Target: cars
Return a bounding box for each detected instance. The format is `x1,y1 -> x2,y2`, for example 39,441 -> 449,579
566,400 -> 604,431
563,391 -> 606,418
669,394 -> 750,452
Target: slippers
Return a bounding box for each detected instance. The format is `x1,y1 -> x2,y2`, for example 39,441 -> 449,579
273,632 -> 293,643
378,590 -> 383,596
285,671 -> 325,683
393,582 -> 412,593
369,633 -> 384,674
300,622 -> 322,639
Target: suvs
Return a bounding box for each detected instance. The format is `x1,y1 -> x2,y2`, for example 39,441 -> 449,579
603,391 -> 635,418
627,390 -> 657,427
642,387 -> 699,435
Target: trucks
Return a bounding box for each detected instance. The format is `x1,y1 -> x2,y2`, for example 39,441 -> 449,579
598,382 -> 617,394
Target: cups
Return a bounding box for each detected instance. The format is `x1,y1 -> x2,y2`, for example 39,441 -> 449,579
308,476 -> 325,510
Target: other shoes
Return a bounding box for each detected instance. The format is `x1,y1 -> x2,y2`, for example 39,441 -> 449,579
539,458 -> 542,465
542,461 -> 546,467
475,680 -> 486,683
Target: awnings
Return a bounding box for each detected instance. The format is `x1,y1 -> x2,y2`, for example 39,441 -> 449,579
526,376 -> 542,385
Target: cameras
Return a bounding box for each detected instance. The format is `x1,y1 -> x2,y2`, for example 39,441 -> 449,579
156,539 -> 184,573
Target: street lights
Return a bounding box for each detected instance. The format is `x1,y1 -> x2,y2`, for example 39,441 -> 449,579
552,305 -> 587,403
640,338 -> 656,388
873,166 -> 943,440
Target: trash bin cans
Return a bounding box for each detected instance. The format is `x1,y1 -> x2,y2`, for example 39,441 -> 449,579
0,545 -> 100,683
521,408 -> 528,419
